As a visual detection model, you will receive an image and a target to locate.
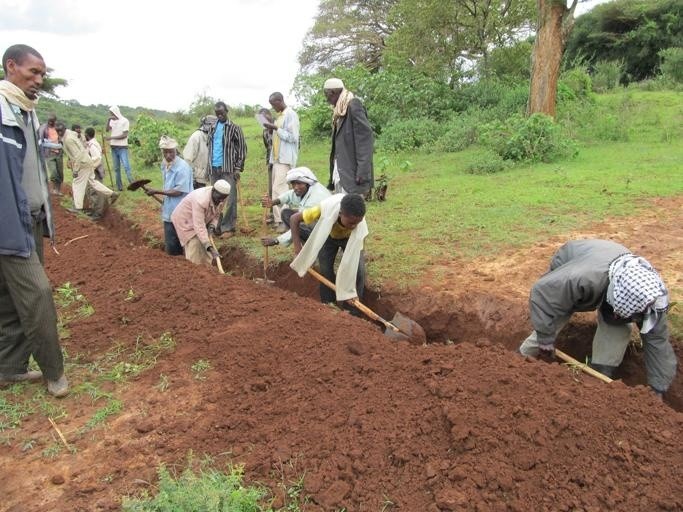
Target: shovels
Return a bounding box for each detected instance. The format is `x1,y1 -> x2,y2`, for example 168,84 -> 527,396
253,192 -> 276,288
308,267 -> 427,347
126,179 -> 162,204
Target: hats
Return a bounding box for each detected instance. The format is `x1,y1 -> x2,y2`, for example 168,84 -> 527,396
324,78 -> 343,88
214,180 -> 231,196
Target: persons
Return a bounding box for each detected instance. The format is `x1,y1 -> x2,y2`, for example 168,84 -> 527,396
323,77 -> 374,200
264,91 -> 299,233
36,102 -> 248,266
262,167 -> 331,249
518,239 -> 677,399
289,193 -> 369,317
259,109 -> 277,224
0,44 -> 69,398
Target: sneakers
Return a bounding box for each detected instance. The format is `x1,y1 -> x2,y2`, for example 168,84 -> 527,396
1,368 -> 44,391
42,374 -> 70,399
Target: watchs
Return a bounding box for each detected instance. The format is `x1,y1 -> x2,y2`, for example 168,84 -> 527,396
274,239 -> 279,244
